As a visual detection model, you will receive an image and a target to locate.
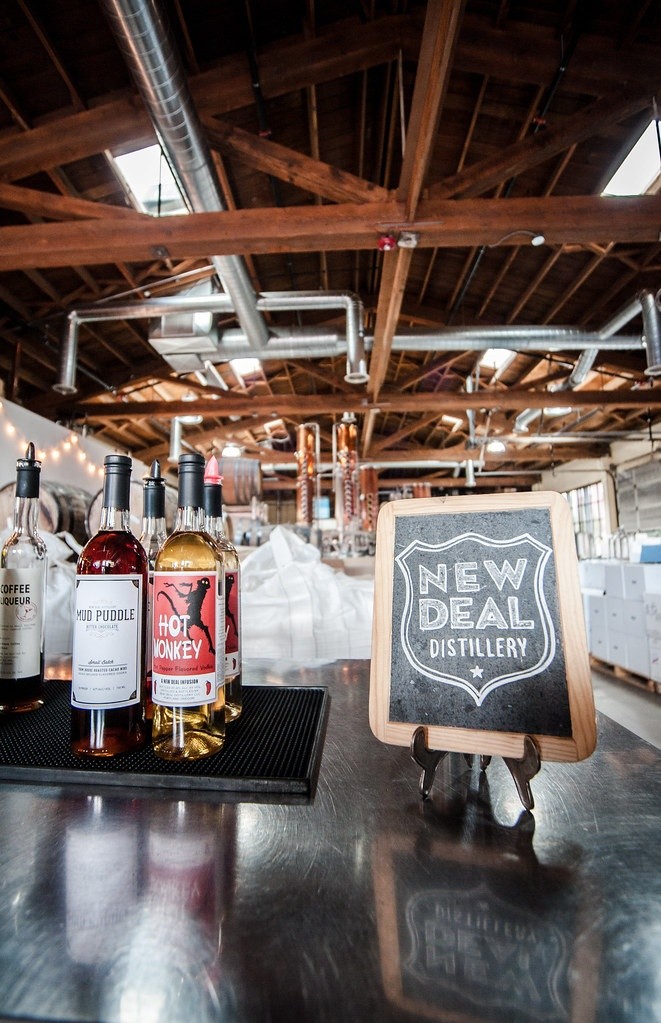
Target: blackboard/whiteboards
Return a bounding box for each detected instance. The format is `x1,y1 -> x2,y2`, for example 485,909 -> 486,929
368,489 -> 599,765
368,824 -> 597,1023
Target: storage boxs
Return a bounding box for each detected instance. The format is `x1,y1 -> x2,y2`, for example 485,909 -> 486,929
588,561 -> 661,681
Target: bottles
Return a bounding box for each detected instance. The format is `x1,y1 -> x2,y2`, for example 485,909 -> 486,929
136,477 -> 169,722
57,791 -> 236,1013
72,454 -> 147,759
154,452 -> 243,763
1,458 -> 47,715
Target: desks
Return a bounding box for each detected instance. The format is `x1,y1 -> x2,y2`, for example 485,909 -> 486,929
0,658 -> 660,1022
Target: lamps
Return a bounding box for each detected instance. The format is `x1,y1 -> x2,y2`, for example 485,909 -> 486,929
489,231 -> 545,248
